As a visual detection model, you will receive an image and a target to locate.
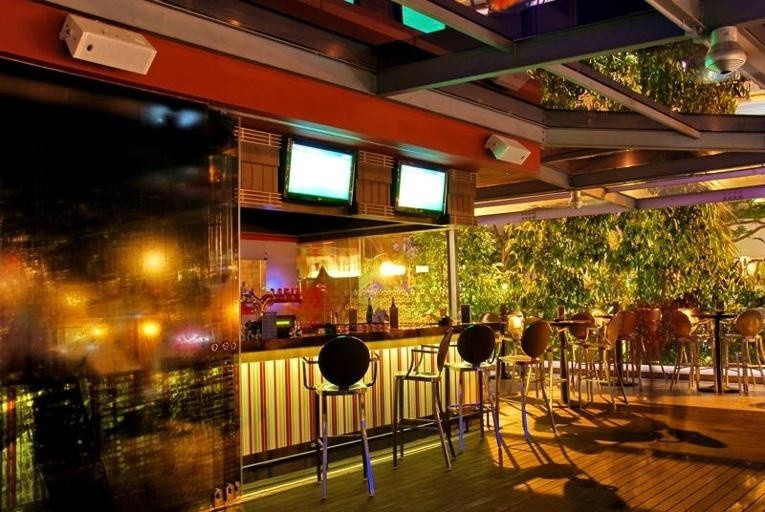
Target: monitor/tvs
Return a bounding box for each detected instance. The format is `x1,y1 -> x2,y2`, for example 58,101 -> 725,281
279,133 -> 358,206
390,156 -> 450,218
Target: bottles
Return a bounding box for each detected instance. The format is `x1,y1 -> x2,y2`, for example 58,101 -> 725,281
366,297 -> 374,322
389,297 -> 398,329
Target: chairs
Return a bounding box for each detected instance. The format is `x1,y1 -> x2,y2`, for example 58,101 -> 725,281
498,321 -> 559,440
303,336 -> 381,500
444,324 -> 500,456
392,327 -> 455,471
480,308 -> 764,411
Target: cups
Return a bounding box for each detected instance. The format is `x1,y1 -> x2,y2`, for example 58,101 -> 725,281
348,308 -> 358,332
557,304 -> 566,321
460,304 -> 471,325
714,299 -> 725,315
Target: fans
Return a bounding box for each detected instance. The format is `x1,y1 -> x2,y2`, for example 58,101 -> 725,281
644,25 -> 765,92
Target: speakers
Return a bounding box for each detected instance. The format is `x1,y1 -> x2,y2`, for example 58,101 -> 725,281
484,133 -> 530,166
59,13 -> 157,78
